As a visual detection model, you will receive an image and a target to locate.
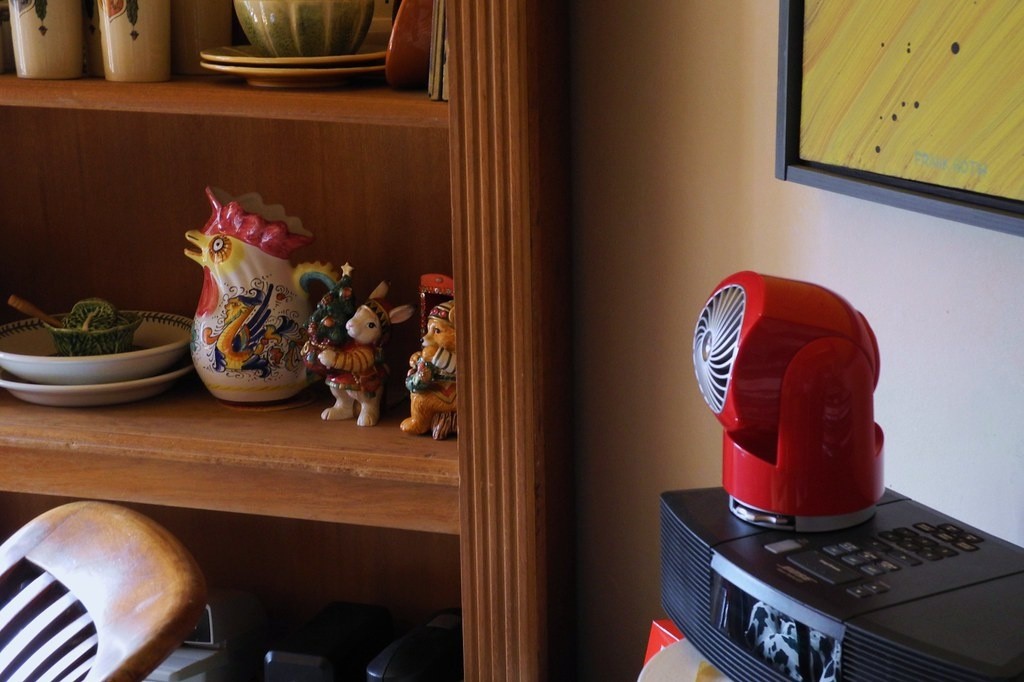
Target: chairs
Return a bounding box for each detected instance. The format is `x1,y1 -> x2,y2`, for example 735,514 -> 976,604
0,501 -> 208,682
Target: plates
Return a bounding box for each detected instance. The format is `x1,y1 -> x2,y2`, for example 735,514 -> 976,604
0,312 -> 196,409
385,0 -> 433,91
198,44 -> 388,88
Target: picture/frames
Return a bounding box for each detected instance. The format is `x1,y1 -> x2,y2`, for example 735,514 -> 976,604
775,0 -> 1024,237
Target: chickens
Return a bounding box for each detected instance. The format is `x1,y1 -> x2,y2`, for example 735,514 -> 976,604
185,184 -> 341,405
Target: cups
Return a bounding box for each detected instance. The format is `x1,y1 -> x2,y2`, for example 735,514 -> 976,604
0,0 -> 234,82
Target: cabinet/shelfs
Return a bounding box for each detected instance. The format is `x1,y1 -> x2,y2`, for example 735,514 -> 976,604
0,0 -> 552,682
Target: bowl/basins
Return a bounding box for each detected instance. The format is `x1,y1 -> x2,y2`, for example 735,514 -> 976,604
232,0 -> 374,56
41,311 -> 142,356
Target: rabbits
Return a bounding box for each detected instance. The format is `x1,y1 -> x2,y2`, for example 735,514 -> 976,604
303,276 -> 415,427
